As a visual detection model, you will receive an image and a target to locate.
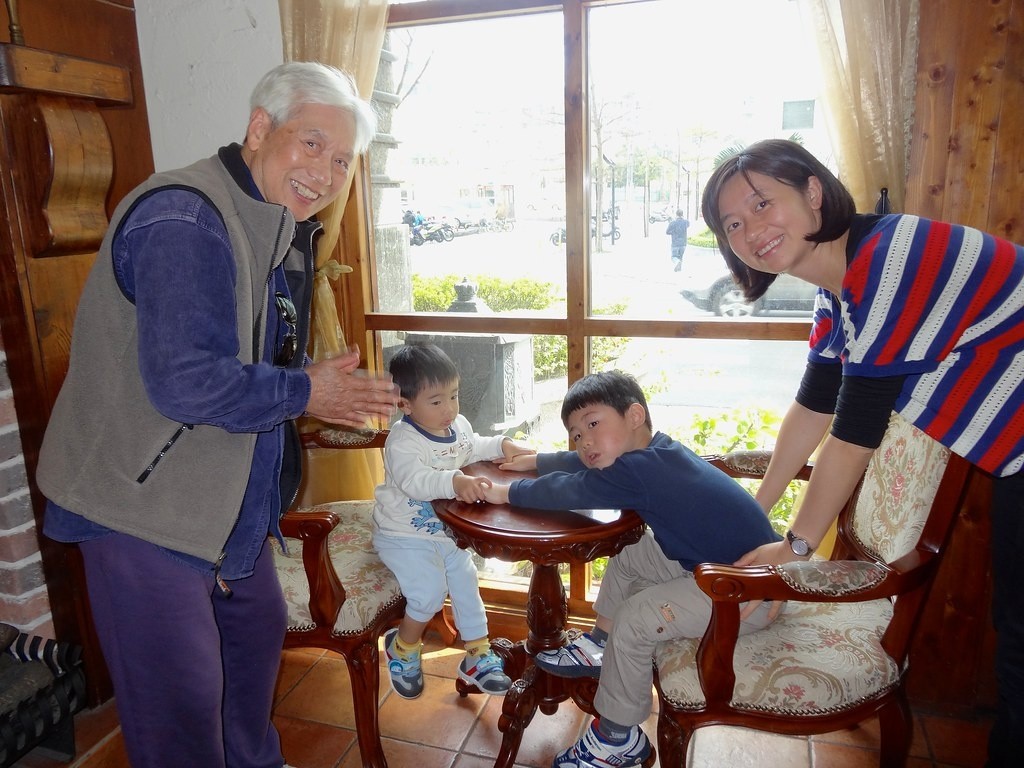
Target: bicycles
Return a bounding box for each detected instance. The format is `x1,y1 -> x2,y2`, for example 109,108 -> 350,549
474,211 -> 515,233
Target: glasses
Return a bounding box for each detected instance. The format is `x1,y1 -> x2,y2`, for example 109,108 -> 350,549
273,291 -> 298,368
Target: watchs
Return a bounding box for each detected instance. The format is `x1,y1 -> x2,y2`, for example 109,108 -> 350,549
788,530 -> 813,556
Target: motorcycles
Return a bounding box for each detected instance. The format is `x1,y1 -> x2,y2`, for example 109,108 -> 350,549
401,210 -> 454,246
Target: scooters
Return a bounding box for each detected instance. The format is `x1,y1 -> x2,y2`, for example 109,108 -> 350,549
549,201 -> 621,246
649,207 -> 672,223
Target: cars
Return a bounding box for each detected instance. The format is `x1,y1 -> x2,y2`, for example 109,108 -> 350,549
707,272 -> 818,317
697,205 -> 704,217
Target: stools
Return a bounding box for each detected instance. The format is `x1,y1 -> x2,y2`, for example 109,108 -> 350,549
0,623 -> 86,767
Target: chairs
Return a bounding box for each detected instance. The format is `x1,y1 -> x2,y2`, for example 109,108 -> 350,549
654,408 -> 986,768
250,409 -> 458,768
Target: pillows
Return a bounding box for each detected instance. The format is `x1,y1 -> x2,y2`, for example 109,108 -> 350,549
0,653 -> 55,713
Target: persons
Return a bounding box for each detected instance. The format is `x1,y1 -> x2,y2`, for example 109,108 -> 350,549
36,64 -> 399,768
702,140 -> 1023,768
667,209 -> 689,272
403,210 -> 424,246
456,372 -> 785,768
374,345 -> 536,697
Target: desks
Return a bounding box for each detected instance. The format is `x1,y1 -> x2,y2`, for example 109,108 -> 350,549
433,459 -> 645,768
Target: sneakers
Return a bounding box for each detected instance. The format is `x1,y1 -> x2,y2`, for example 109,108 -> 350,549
456,649 -> 513,695
535,633 -> 605,678
383,628 -> 424,699
554,718 -> 651,768
